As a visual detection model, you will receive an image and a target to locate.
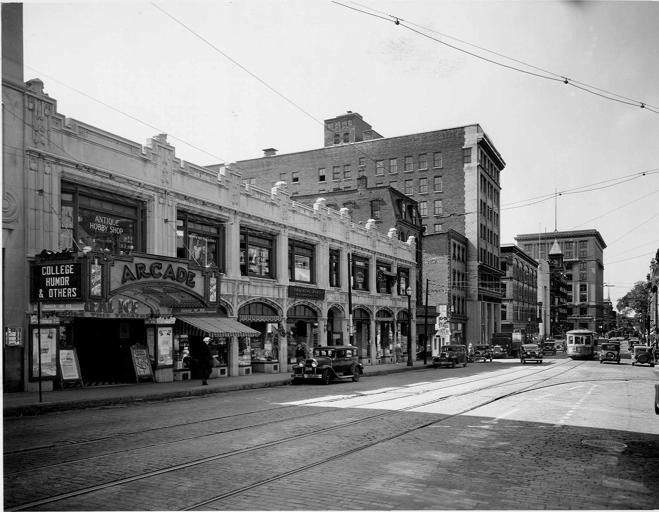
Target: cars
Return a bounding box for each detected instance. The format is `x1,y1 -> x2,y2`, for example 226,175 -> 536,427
519,343 -> 543,364
593,337 -> 622,365
289,344 -> 365,385
627,339 -> 656,367
428,344 -> 509,370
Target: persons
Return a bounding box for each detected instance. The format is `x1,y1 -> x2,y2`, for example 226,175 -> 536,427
296,343 -> 306,365
197,336 -> 215,385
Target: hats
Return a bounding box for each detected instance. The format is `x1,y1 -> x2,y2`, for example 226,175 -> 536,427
203,337 -> 211,342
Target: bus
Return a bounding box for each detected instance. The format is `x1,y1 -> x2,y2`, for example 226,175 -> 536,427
565,326 -> 594,361
